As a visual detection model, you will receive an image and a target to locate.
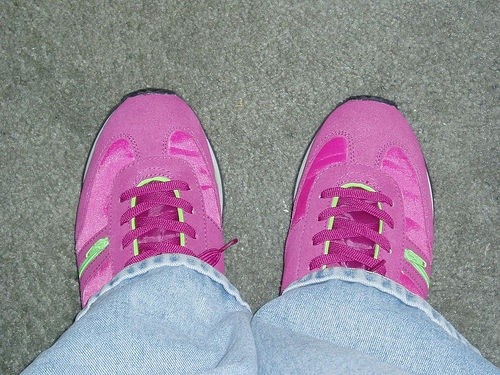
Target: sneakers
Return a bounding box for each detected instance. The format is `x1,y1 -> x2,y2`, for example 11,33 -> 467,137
73,87 -> 239,312
279,96 -> 434,311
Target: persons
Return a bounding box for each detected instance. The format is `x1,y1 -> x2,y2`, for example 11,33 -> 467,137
17,87 -> 500,375
